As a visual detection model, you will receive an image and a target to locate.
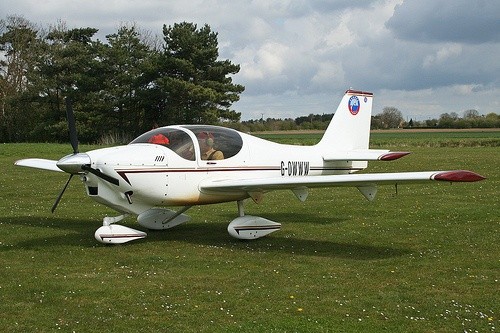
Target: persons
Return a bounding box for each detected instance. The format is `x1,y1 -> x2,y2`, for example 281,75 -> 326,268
189,130 -> 225,161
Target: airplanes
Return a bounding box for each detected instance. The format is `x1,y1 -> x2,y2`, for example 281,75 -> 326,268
14,86 -> 486,244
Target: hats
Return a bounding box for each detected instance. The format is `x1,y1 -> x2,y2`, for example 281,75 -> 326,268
197,130 -> 215,147
